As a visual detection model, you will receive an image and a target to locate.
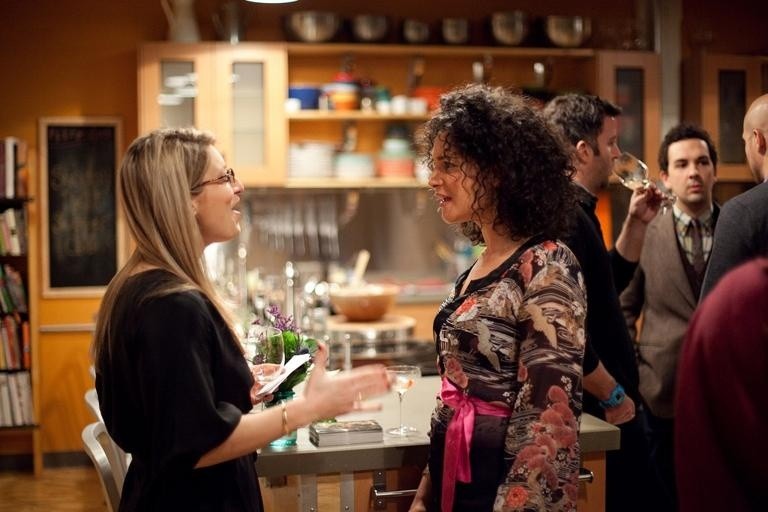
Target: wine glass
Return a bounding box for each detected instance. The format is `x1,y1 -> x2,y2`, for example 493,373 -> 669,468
610,151 -> 678,215
247,324 -> 285,412
382,365 -> 422,436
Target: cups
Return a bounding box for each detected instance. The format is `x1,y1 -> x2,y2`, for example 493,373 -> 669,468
210,0 -> 248,41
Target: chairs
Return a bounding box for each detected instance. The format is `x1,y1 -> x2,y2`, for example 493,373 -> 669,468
80,363 -> 131,512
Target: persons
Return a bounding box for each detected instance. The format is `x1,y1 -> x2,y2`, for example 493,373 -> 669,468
617,124 -> 720,453
93,129 -> 393,509
405,81 -> 589,512
699,93 -> 767,301
544,92 -> 672,511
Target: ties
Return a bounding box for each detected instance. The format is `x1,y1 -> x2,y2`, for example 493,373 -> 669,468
690,218 -> 706,289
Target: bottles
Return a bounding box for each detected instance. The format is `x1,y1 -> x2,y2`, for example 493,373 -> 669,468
161,0 -> 202,42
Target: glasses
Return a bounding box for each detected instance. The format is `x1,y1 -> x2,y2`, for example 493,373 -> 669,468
191,168 -> 236,190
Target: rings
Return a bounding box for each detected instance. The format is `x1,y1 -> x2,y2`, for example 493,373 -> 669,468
358,390 -> 362,401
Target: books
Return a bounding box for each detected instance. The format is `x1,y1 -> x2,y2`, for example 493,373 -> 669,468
1,133 -> 37,430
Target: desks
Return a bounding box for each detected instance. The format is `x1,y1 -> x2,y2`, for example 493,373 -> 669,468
255,372 -> 623,512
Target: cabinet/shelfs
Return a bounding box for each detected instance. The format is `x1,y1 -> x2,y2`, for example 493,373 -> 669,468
597,42 -> 664,245
680,42 -> 768,209
0,149 -> 39,475
135,40 -> 289,187
286,40 -> 597,193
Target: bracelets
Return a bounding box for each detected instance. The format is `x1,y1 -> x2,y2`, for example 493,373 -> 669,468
278,398 -> 290,437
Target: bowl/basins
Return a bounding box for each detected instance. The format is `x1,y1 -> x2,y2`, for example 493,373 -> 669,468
489,9 -> 531,47
440,16 -> 470,46
286,7 -> 340,43
544,12 -> 592,47
401,17 -> 431,44
328,285 -> 399,322
289,87 -> 322,110
351,15 -> 391,44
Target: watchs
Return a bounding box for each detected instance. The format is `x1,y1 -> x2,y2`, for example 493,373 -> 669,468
599,385 -> 624,409
